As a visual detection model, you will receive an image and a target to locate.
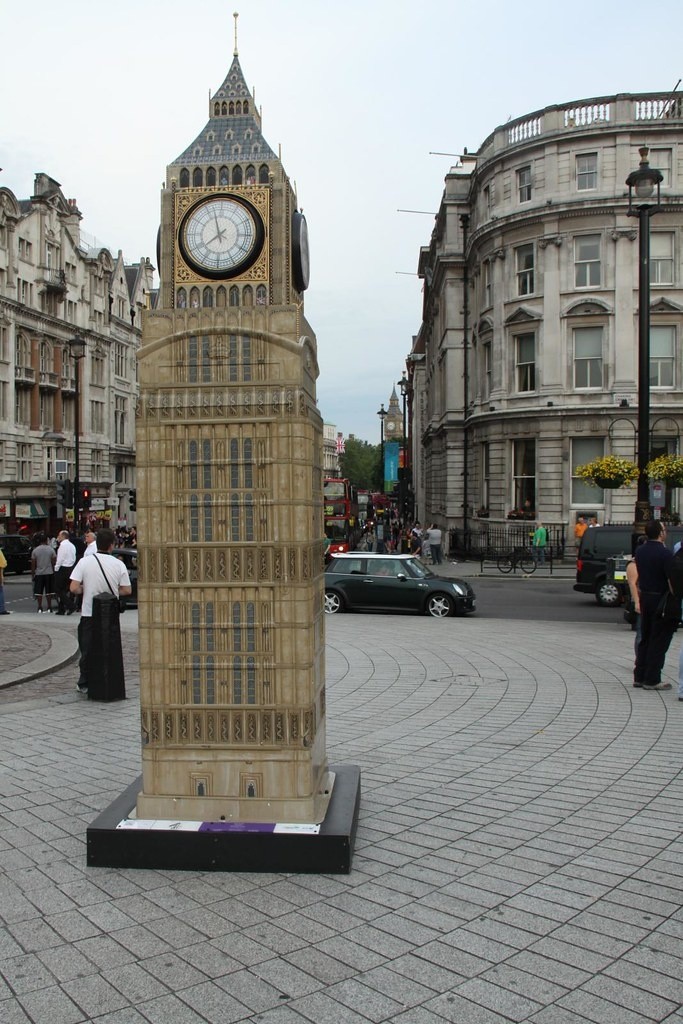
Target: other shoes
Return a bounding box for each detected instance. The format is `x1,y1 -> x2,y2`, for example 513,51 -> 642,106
37,608 -> 43,613
55,611 -> 64,615
0,610 -> 10,615
633,680 -> 643,687
67,605 -> 78,615
77,684 -> 88,693
47,608 -> 52,613
643,682 -> 672,690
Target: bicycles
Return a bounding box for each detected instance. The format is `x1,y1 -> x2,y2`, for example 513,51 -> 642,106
497,546 -> 537,574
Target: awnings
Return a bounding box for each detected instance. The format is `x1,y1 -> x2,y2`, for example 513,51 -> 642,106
17,499 -> 49,519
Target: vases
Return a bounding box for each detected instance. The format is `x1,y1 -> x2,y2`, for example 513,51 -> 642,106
594,477 -> 624,489
508,515 -> 535,520
477,513 -> 489,517
667,478 -> 683,489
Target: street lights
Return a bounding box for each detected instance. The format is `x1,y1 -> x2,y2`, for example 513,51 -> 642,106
377,404 -> 388,495
66,334 -> 88,544
397,371 -> 412,523
626,145 -> 665,521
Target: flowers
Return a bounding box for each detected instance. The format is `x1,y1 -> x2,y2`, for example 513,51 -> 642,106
575,453 -> 639,490
475,508 -> 489,514
509,508 -> 535,516
642,454 -> 683,489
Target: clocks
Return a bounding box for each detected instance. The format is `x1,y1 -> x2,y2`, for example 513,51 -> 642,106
293,213 -> 310,290
400,422 -> 403,431
179,195 -> 264,279
386,422 -> 395,431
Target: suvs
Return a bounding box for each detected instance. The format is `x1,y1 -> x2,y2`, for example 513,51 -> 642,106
572,524 -> 683,606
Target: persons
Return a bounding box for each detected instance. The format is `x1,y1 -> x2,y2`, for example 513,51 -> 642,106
0,526 -> 137,616
588,517 -> 600,527
625,519 -> 683,701
69,530 -> 132,694
574,517 -> 588,561
365,506 -> 443,565
531,522 -> 547,565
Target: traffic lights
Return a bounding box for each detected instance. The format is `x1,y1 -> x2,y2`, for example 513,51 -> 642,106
57,479 -> 73,509
81,487 -> 91,508
129,489 -> 136,512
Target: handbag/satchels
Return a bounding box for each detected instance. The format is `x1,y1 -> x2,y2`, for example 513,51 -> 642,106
118,599 -> 126,613
624,607 -> 637,624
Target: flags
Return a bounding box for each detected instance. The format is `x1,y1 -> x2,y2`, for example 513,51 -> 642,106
336,438 -> 345,454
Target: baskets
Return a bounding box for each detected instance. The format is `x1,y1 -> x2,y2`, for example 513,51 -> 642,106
594,474 -> 625,488
667,474 -> 683,487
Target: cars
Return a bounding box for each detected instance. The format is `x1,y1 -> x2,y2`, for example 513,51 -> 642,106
0,534 -> 33,572
325,551 -> 478,619
110,549 -> 138,604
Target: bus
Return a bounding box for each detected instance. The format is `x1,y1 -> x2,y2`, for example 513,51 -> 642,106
323,477 -> 359,562
358,491 -> 387,530
